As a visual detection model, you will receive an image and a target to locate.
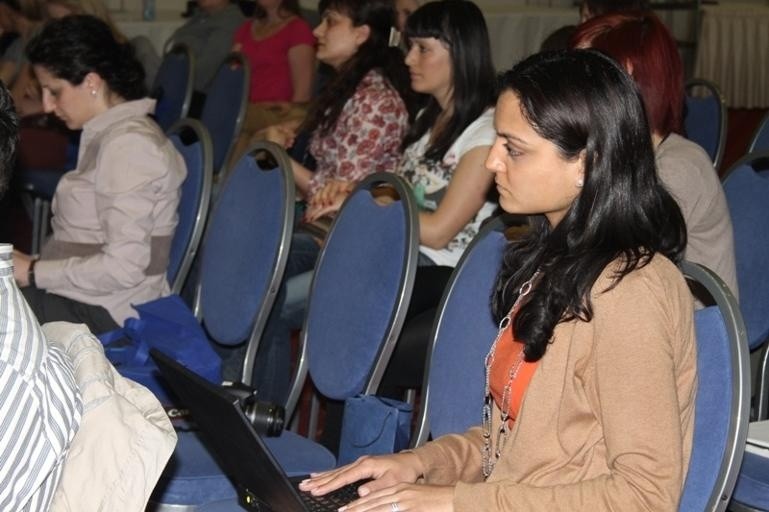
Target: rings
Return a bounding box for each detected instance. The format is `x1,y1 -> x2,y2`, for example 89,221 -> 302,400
389,501 -> 402,512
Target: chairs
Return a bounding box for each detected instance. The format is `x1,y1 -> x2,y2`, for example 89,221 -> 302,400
15,37 -> 768,511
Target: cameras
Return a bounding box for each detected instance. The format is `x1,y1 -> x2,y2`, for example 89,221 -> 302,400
217,380 -> 286,437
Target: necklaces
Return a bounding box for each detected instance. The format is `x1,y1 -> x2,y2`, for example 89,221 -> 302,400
475,254 -> 562,482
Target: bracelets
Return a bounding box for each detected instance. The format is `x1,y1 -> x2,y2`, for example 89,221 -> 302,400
23,251 -> 43,292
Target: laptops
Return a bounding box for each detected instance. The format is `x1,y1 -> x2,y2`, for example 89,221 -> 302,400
150,348 -> 376,512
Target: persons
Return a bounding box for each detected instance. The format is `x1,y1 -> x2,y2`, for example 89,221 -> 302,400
0,0 -> 658,215
1,16 -> 189,335
249,0 -> 407,409
310,1 -> 494,457
570,9 -> 739,322
0,235 -> 83,509
298,48 -> 695,510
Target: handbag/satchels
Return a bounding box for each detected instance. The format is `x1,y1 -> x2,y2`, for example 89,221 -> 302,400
218,100 -> 311,178
302,187 -> 397,246
339,394 -> 414,466
